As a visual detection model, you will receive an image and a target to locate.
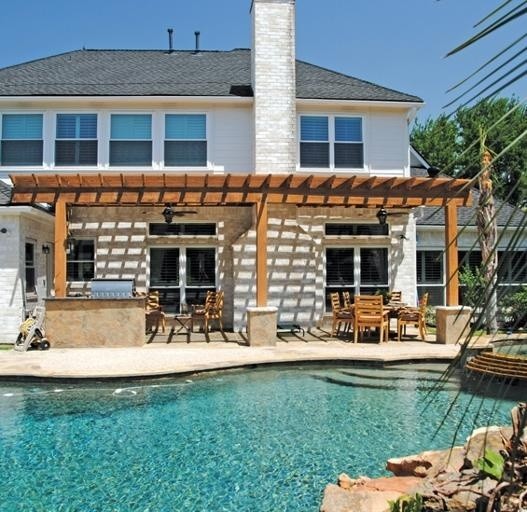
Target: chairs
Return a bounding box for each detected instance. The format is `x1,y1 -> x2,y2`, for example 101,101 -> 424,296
133,290 -> 224,336
331,291 -> 429,343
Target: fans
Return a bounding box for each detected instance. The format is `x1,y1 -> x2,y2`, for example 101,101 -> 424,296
142,206 -> 197,224
364,205 -> 409,226
